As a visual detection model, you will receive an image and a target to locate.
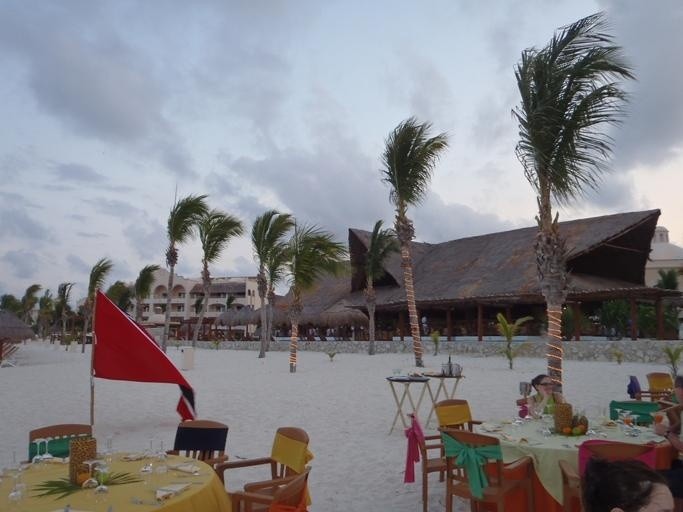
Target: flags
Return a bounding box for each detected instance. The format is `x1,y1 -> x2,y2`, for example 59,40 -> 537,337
91,289 -> 195,421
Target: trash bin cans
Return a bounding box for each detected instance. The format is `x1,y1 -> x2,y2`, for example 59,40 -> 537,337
65,334 -> 71,345
85,335 -> 92,344
71,339 -> 78,352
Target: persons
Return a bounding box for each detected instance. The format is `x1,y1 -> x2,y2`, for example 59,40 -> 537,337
527,374 -> 562,419
582,457 -> 675,512
654,377 -> 683,497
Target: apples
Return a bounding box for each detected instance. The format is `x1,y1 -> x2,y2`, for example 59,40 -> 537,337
572,427 -> 582,436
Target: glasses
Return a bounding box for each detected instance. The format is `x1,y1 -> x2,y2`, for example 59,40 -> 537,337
540,382 -> 553,385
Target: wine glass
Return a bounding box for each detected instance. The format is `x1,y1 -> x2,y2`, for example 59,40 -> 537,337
8,436 -> 167,504
512,405 -> 640,441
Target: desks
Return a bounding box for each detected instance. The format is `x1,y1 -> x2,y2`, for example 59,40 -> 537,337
421,370 -> 465,428
386,375 -> 429,439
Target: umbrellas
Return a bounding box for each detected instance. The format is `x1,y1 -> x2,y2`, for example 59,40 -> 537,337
213,299 -> 369,330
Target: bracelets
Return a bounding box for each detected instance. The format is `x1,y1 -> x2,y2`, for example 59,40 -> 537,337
663,430 -> 670,438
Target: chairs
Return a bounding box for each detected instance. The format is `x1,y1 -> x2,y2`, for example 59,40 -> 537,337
214,426 -> 312,512
0,343 -> 19,368
165,420 -> 229,473
438,427 -> 534,512
410,411 -> 466,512
647,373 -> 675,401
433,399 -> 485,481
517,399 -> 529,418
630,375 -> 671,403
30,423 -> 93,464
232,465 -> 311,512
607,400 -> 662,428
